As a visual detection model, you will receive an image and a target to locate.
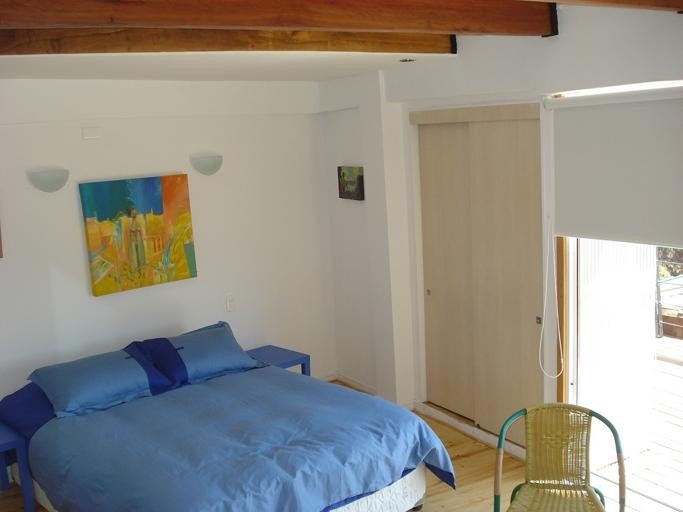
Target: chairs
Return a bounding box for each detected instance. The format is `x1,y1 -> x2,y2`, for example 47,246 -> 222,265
494,403 -> 625,512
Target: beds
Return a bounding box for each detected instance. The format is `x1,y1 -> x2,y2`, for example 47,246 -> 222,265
0,364 -> 426,511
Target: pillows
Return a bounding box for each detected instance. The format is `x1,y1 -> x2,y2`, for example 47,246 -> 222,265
27,340 -> 180,418
137,322 -> 270,389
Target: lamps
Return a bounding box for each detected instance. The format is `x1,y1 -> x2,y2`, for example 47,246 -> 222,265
192,155 -> 222,175
30,169 -> 69,192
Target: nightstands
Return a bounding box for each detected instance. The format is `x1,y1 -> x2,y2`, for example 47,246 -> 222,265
0,421 -> 35,512
247,344 -> 310,377
